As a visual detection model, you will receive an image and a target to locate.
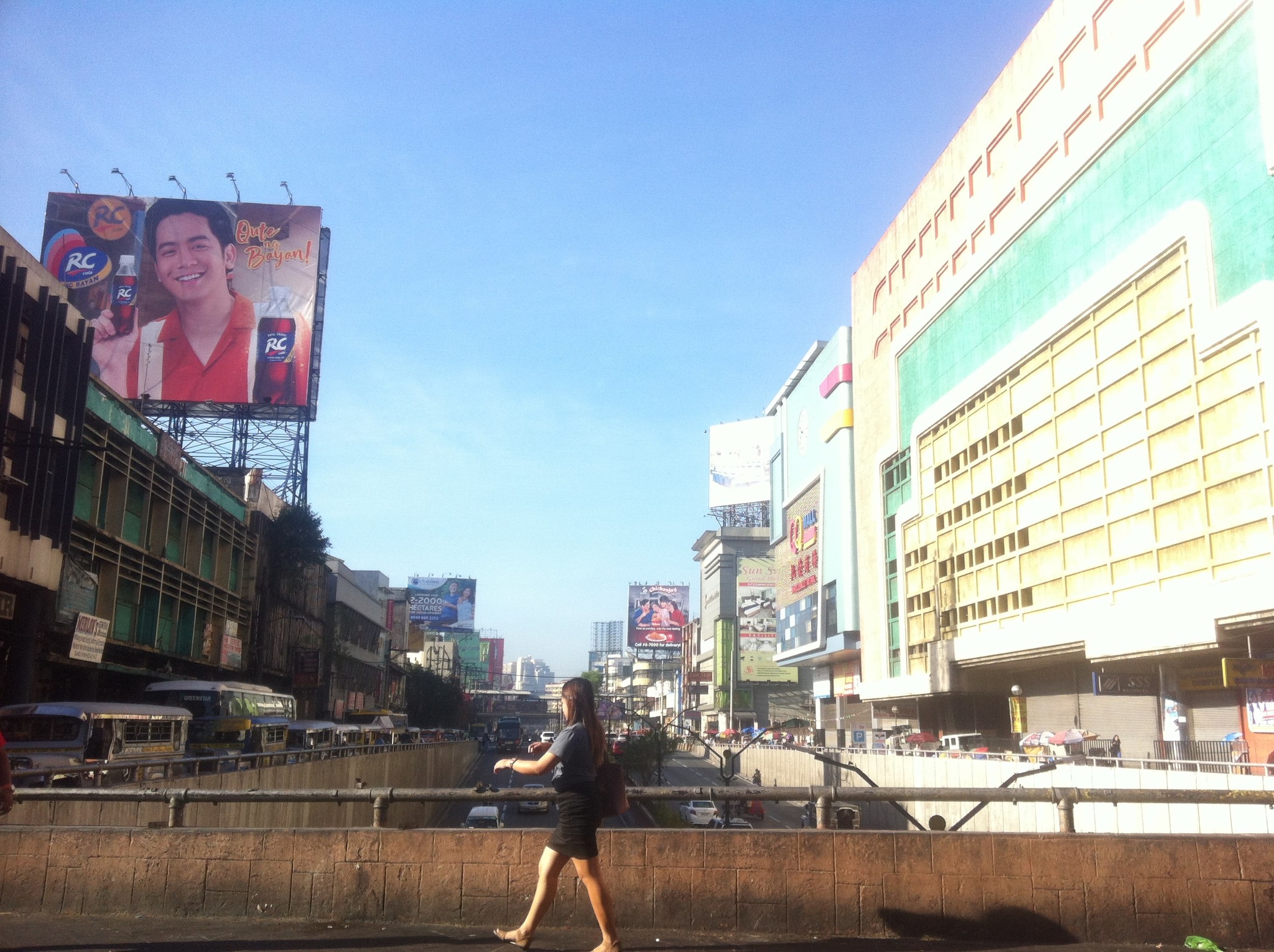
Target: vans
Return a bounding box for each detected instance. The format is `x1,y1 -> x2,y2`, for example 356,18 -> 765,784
680,736 -> 694,745
460,807 -> 504,830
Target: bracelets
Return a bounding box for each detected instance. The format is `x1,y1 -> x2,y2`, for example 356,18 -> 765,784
503,760 -> 509,768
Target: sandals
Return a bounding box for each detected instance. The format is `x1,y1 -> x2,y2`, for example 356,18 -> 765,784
493,929 -> 534,951
590,939 -> 622,952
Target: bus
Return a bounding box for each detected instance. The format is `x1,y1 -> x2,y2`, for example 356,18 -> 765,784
183,716 -> 291,775
331,723 -> 470,758
469,722 -> 488,743
0,701 -> 193,789
346,709 -> 409,746
137,679 -> 297,724
495,716 -> 524,754
284,719 -> 338,765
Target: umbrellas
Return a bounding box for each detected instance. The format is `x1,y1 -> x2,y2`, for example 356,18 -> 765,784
1019,730 -> 1057,746
906,733 -> 940,749
703,726 -> 791,745
964,747 -> 990,760
1048,728 -> 1099,755
935,747 -> 967,758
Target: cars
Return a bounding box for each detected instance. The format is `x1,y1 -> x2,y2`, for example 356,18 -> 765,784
679,799 -> 720,826
518,784 -> 548,814
883,733 -> 985,756
605,730 -> 647,756
487,734 -> 497,743
522,730 -> 558,744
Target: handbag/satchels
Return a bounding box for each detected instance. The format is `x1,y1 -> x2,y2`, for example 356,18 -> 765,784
598,762 -> 630,818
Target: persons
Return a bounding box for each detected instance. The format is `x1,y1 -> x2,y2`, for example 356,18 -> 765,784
1110,734 -> 1123,767
418,581 -> 475,630
752,769 -> 761,786
371,730 -> 415,753
1037,745 -> 1049,763
792,737 -> 923,756
491,677 -> 621,952
633,595 -> 686,629
88,197 -> 310,407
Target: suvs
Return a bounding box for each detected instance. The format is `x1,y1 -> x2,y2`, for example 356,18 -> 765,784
707,817 -> 754,829
800,800 -> 862,831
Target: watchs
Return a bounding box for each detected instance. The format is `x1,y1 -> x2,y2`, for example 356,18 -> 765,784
509,757 -> 518,771
